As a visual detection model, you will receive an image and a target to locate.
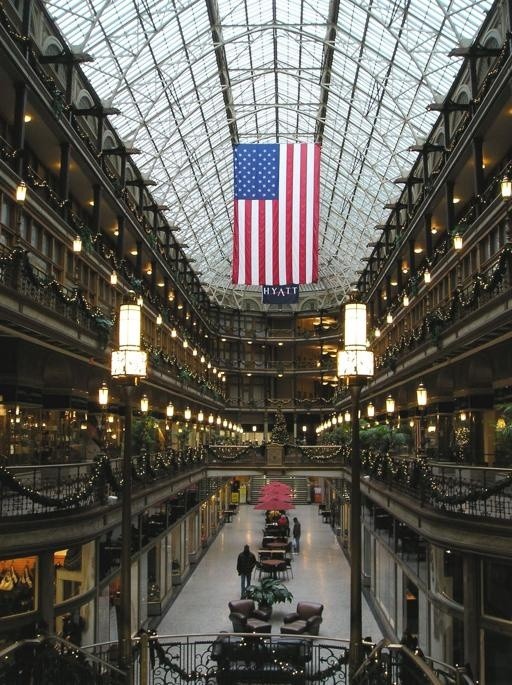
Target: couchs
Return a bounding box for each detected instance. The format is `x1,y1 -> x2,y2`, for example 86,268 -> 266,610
212,631 -> 312,684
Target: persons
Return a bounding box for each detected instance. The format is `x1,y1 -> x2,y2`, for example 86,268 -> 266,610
293,518 -> 302,553
236,544 -> 257,599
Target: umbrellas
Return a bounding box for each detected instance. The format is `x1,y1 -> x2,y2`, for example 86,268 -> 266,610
255,481 -> 295,522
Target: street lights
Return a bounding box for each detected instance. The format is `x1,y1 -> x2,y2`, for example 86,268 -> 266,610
97,379 -> 110,506
415,381 -> 429,463
251,424 -> 257,447
166,400 -> 175,480
315,408 -> 351,447
140,393 -> 149,490
336,299 -> 374,683
197,408 -> 244,464
183,405 -> 192,472
366,400 -> 375,450
111,304 -> 148,685
303,426 -> 308,446
385,393 -> 395,455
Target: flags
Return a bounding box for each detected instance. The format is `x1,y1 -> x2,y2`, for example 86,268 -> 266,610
232,142 -> 321,286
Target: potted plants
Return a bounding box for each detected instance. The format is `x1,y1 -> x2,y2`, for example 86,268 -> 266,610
243,578 -> 293,618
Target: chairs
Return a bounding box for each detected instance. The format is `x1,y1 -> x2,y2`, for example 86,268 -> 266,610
253,510 -> 294,582
228,600 -> 272,634
281,601 -> 324,635
